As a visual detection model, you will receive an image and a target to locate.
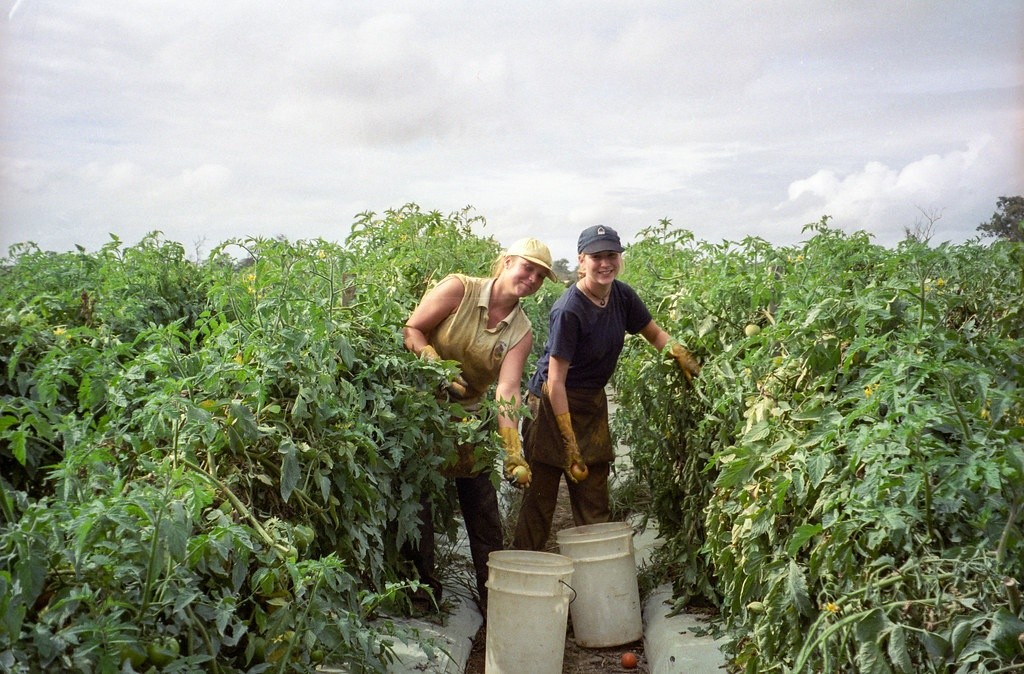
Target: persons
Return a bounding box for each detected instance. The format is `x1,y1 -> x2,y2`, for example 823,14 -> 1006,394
400,239 -> 558,627
513,225 -> 701,552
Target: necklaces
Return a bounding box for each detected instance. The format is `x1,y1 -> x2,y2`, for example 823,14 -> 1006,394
584,277 -> 612,306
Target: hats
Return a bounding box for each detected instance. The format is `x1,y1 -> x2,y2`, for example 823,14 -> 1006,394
507,236 -> 557,283
578,225 -> 625,255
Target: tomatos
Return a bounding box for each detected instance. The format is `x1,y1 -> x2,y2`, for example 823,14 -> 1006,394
622,653 -> 637,668
511,465 -> 529,483
571,462 -> 588,481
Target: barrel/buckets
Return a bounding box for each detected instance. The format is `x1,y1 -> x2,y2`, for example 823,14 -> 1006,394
555,522 -> 644,649
484,550 -> 577,674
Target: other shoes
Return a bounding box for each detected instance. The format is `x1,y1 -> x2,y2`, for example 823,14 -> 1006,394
478,600 -> 487,620
407,588 -> 431,616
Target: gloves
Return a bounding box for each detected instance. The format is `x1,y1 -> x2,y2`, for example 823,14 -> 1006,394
498,427 -> 533,488
419,344 -> 469,402
667,343 -> 701,382
555,413 -> 585,485
